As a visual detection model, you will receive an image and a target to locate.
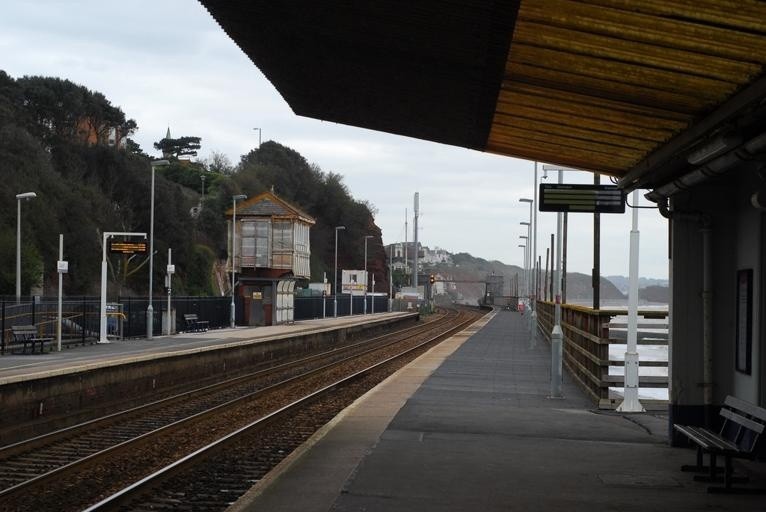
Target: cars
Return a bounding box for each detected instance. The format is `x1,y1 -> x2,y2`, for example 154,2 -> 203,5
0,302 -> 124,339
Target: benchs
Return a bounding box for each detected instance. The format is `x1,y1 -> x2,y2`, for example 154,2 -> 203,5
671,395 -> 765,500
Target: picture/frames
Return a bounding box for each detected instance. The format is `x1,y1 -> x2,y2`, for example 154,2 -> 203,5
731,265 -> 753,377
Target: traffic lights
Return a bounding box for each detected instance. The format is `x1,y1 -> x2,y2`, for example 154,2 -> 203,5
429,274 -> 434,284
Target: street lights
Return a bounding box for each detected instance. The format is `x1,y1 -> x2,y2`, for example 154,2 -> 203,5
227,193 -> 248,328
514,195 -> 533,325
197,171 -> 207,200
333,226 -> 345,319
11,189 -> 38,302
253,127 -> 262,149
361,234 -> 376,316
144,158 -> 170,342
